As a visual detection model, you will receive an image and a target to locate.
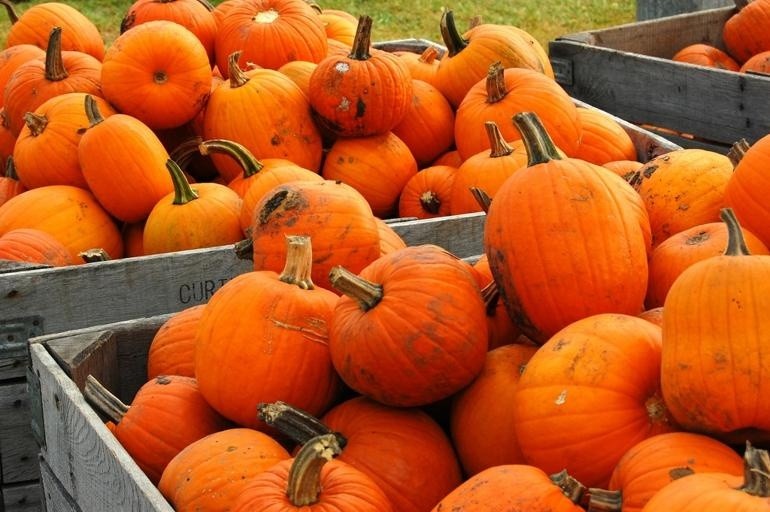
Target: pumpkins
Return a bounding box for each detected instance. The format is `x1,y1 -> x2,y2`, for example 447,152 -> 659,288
0,0 -> 635,268
641,0 -> 770,140
84,129 -> 770,512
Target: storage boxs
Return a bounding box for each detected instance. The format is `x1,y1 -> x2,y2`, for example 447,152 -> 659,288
31,253 -> 490,512
548,2 -> 770,152
1,37 -> 684,510
